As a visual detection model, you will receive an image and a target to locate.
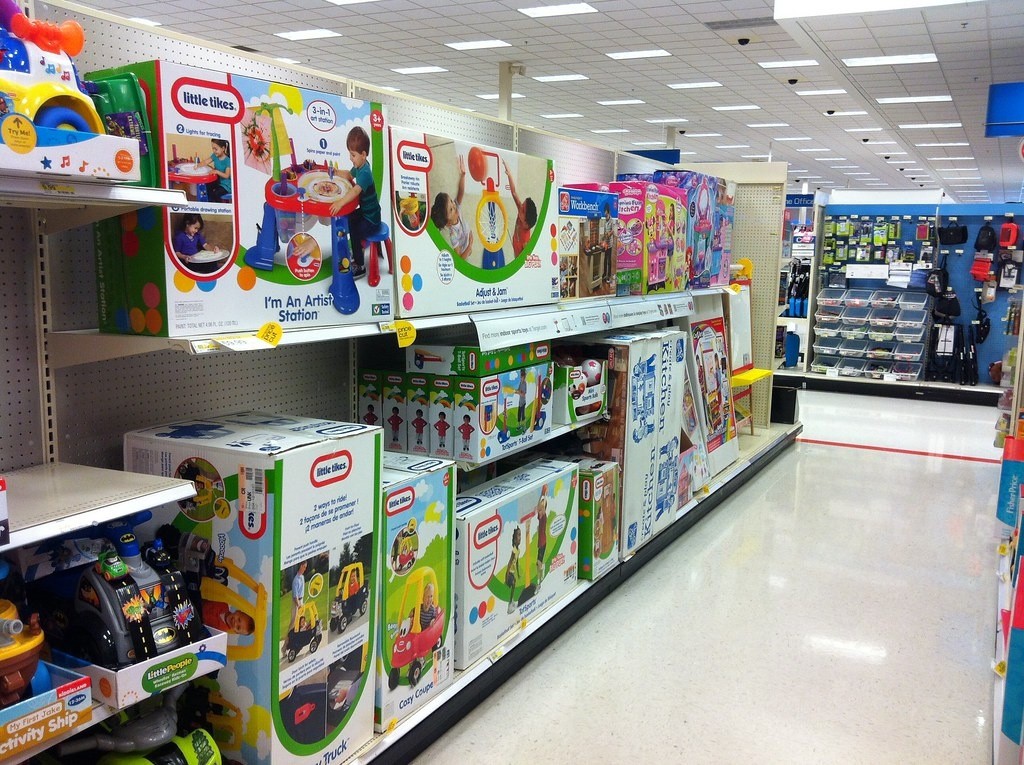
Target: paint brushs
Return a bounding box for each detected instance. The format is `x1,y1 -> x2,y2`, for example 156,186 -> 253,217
329,160 -> 334,182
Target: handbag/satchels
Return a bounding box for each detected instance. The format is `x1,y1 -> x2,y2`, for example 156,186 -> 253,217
925,254 -> 949,297
935,287 -> 960,320
938,220 -> 968,245
974,223 -> 997,251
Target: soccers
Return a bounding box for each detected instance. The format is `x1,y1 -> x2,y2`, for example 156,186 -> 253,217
580,359 -> 602,387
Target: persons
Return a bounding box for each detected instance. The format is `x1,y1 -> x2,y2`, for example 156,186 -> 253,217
349,571 -> 359,596
515,367 -> 526,432
602,202 -> 615,282
696,355 -> 714,434
505,526 -> 521,614
406,583 -> 437,677
173,214 -> 219,275
610,490 -> 616,541
328,125 -> 381,280
335,674 -> 363,706
501,159 -> 538,259
299,616 -> 310,631
534,490 -> 548,595
194,139 -> 232,203
392,531 -> 406,571
665,204 -> 675,283
363,404 -> 475,451
714,353 -> 724,429
203,600 -> 255,636
432,154 -> 474,262
281,561 -> 308,657
597,508 -> 604,555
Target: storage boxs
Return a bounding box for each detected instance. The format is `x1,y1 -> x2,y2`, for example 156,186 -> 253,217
1,111 -> 141,183
673,295 -> 740,478
124,409 -> 386,765
559,169 -> 738,301
390,126 -> 564,320
356,301 -> 705,735
16,533 -> 229,711
810,288 -> 930,381
0,662 -> 94,762
83,62 -> 395,338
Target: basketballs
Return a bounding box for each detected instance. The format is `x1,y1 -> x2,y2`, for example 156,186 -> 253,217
468,147 -> 487,181
542,484 -> 548,496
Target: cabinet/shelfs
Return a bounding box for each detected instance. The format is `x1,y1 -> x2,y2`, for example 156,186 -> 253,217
770,197 -> 1024,465
352,78 -> 627,711
517,125 -> 706,584
0,0 -> 228,765
613,149 -> 806,514
29,0 -> 500,765
993,298 -> 1024,765
